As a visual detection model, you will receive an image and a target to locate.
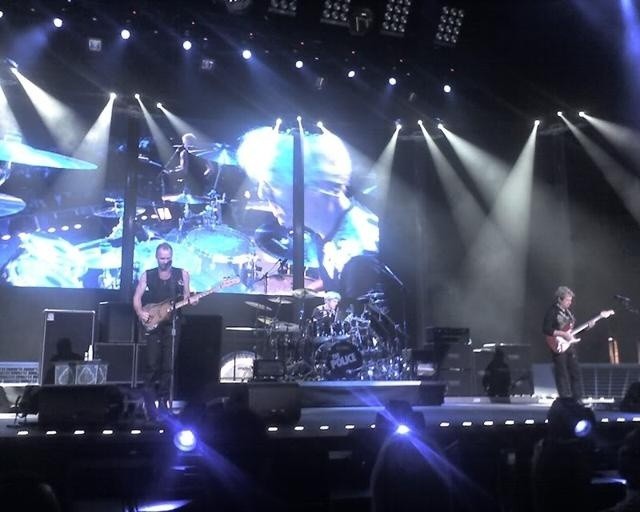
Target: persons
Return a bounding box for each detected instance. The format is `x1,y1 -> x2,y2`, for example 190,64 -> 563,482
132,242 -> 199,397
235,122 -> 380,291
1,399 -> 640,511
541,286 -> 582,406
311,291 -> 341,321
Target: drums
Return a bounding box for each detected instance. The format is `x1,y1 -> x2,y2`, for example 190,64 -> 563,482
269,322 -> 300,349
178,201 -> 254,257
245,275 -> 315,295
307,316 -> 369,379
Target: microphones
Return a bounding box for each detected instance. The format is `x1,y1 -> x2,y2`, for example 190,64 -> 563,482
369,256 -> 404,288
356,292 -> 384,304
615,294 -> 631,303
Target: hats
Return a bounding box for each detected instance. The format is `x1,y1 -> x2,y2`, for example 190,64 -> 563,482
324,291 -> 341,301
246,127 -> 353,185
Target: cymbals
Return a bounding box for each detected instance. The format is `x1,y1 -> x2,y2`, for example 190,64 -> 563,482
94,208 -> 146,218
255,223 -> 295,262
357,293 -> 384,301
246,301 -> 272,311
257,317 -> 280,328
0,193 -> 25,217
185,142 -> 239,165
269,298 -> 292,304
161,195 -> 210,206
0,140 -> 98,170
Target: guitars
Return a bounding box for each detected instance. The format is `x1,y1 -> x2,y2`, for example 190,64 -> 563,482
141,276 -> 240,331
546,309 -> 615,354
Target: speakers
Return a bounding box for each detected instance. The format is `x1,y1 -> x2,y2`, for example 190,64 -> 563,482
94,301 -> 223,385
41,309 -> 96,384
